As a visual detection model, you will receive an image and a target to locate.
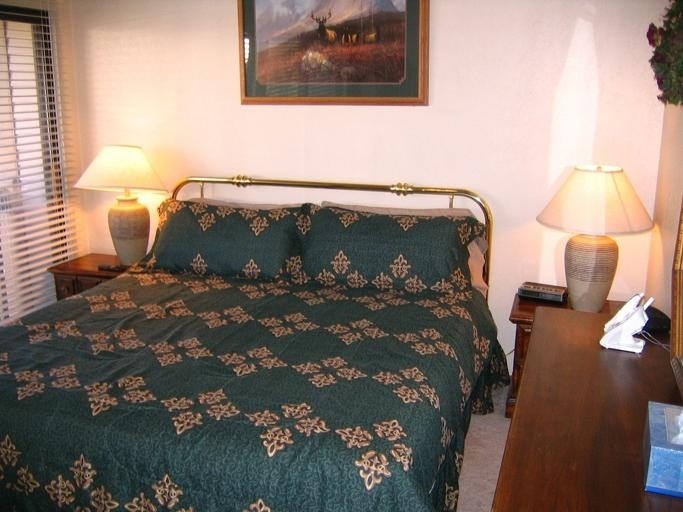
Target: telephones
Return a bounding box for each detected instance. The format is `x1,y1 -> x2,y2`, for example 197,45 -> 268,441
599,292 -> 656,355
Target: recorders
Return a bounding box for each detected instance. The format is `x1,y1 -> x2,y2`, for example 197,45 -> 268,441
517,284 -> 566,302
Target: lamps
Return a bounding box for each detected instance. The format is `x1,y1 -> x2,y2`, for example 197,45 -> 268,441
75,144 -> 168,265
534,164 -> 654,312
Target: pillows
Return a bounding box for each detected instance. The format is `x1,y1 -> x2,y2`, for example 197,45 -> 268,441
128,197 -> 317,296
312,206 -> 485,299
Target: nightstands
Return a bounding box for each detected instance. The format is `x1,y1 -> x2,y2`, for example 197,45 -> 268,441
48,253 -> 126,302
506,299 -> 622,419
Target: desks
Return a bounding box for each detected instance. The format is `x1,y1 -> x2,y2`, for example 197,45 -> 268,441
491,306 -> 683,512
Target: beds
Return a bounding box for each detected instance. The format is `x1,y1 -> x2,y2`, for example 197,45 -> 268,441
0,176 -> 509,512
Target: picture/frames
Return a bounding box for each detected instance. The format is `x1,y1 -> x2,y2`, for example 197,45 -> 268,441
236,1 -> 431,106
